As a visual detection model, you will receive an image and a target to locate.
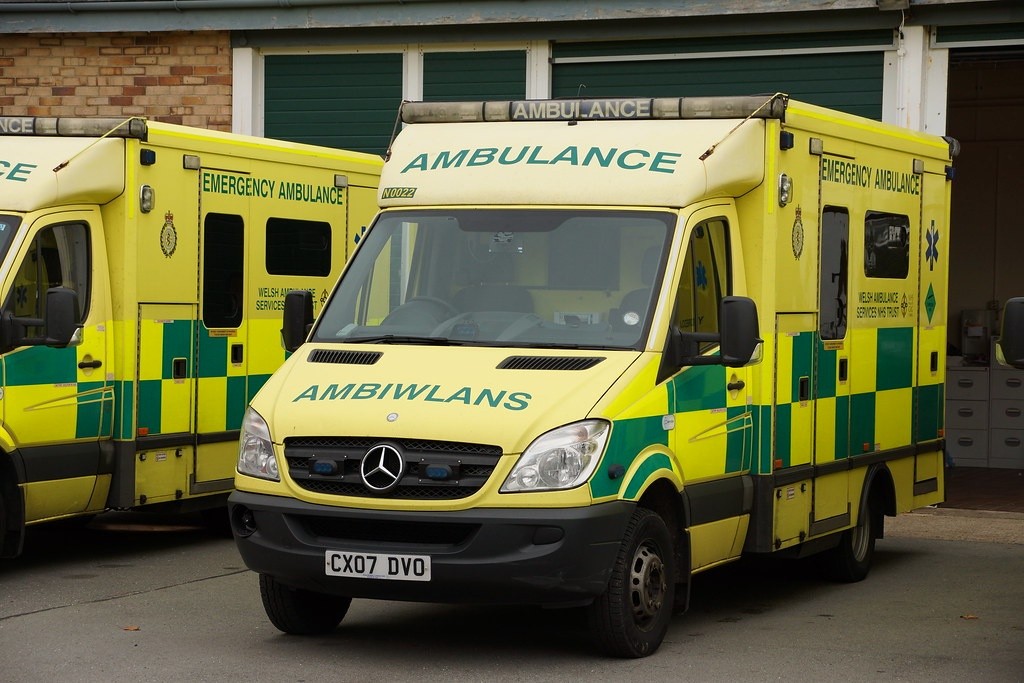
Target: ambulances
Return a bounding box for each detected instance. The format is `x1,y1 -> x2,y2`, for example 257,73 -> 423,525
0,113 -> 422,568
226,93 -> 950,660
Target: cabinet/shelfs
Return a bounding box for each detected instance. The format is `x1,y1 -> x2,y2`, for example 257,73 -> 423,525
947,335 -> 1024,470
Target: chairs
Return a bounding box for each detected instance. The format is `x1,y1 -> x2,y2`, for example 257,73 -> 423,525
455,285 -> 534,315
617,287 -> 651,337
